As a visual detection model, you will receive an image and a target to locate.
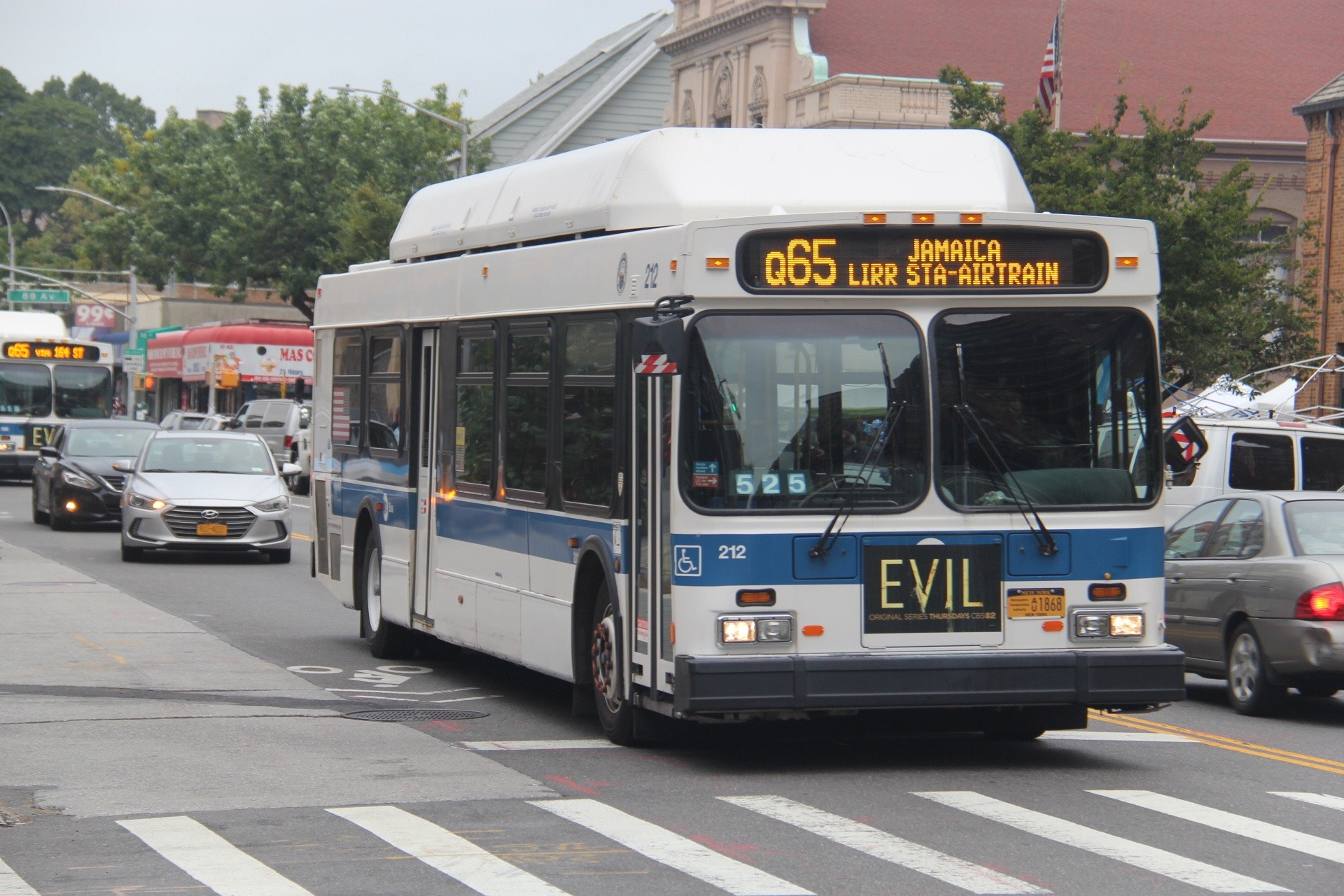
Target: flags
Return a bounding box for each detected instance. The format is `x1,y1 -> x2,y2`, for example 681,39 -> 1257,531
1033,13 -> 1064,121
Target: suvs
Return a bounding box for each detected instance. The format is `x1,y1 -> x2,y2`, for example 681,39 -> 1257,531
159,409 -> 211,431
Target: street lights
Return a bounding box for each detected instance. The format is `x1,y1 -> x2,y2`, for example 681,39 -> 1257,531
35,185 -> 137,421
327,82 -> 467,176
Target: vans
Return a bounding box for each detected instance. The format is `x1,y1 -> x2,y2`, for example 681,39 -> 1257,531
1099,416 -> 1344,552
222,399 -> 312,480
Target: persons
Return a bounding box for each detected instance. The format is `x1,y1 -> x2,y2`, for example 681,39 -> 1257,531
803,418 -> 883,473
965,364 -> 1077,472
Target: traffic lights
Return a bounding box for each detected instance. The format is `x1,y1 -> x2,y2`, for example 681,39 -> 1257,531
144,376 -> 158,390
205,371 -> 211,386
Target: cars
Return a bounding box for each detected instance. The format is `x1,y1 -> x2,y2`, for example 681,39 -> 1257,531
30,418 -> 159,532
285,428 -> 310,495
1162,490 -> 1344,716
112,429 -> 302,566
196,414 -> 235,432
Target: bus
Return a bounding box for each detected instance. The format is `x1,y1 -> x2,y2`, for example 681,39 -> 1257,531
310,127 -> 1209,754
0,310 -> 121,482
129,319 -> 315,425
